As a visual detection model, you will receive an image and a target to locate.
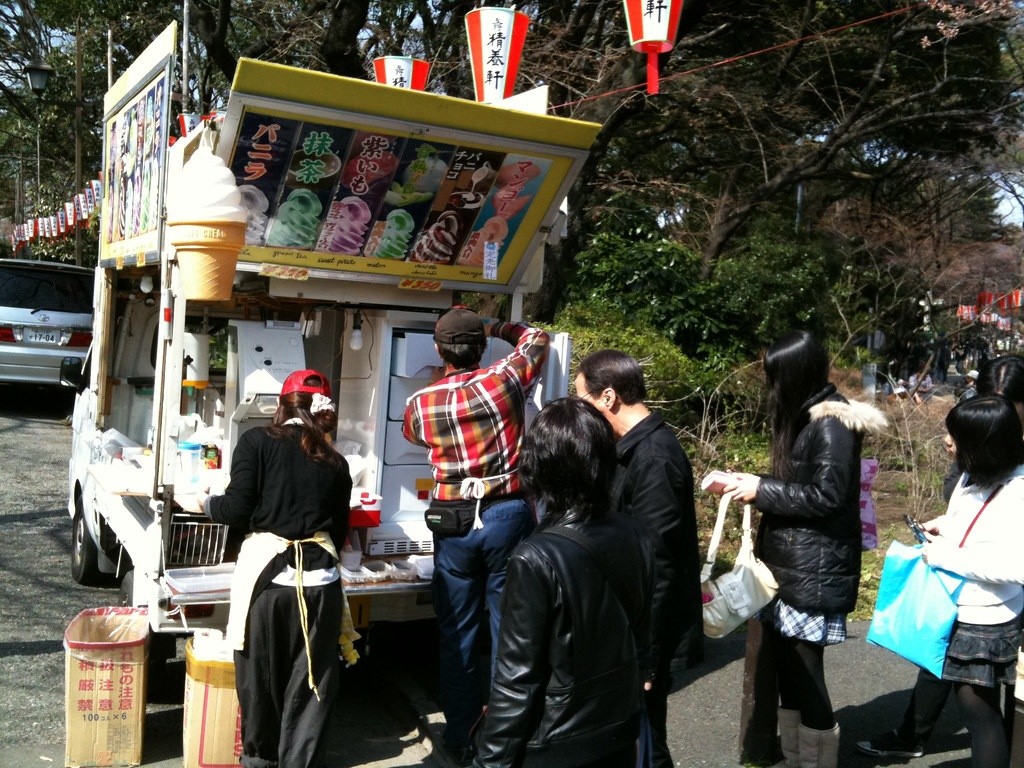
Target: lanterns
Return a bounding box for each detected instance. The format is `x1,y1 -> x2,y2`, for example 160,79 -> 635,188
621,0 -> 683,95
167,110 -> 226,147
464,7 -> 530,103
956,289 -> 1024,331
10,180 -> 103,251
373,56 -> 430,92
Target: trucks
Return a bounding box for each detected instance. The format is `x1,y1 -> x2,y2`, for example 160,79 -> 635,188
60,118 -> 572,703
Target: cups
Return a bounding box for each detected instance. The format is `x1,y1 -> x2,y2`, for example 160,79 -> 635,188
362,494 -> 383,511
340,551 -> 362,571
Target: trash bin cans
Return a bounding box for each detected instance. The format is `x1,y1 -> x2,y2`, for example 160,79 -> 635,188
181,637 -> 243,768
62,607 -> 150,766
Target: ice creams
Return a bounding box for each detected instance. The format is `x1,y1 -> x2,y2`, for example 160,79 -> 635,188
237,186 -> 509,267
104,157 -> 159,240
165,137 -> 249,302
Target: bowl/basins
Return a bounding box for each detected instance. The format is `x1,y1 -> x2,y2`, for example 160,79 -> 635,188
337,560 -> 417,583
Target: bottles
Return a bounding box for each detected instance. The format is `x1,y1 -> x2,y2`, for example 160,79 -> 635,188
353,531 -> 361,552
178,444 -> 201,493
344,537 -> 353,552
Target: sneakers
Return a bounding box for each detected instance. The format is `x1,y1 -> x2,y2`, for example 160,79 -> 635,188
856,732 -> 925,760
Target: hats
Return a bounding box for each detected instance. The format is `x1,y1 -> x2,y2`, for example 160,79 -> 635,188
435,308 -> 487,344
967,370 -> 979,380
280,370 -> 331,399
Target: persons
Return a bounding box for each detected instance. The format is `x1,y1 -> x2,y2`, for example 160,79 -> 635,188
899,317 -> 1024,399
401,308 -> 551,768
471,398 -> 687,768
574,350 -> 704,768
197,370 -> 352,768
724,328 -> 888,768
858,353 -> 1024,768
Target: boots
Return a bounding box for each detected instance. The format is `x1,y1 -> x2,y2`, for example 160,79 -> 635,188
768,706 -> 841,768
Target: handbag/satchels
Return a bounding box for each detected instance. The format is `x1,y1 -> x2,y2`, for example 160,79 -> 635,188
868,540 -> 965,683
859,455 -> 880,552
702,493 -> 781,641
425,501 -> 487,538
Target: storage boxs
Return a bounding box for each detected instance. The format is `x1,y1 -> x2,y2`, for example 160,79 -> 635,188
63,607 -> 149,768
182,636 -> 242,768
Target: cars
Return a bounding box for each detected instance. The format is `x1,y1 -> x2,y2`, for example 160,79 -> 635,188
0,259 -> 95,421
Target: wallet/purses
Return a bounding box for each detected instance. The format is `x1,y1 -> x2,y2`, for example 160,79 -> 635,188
700,470 -> 737,495
